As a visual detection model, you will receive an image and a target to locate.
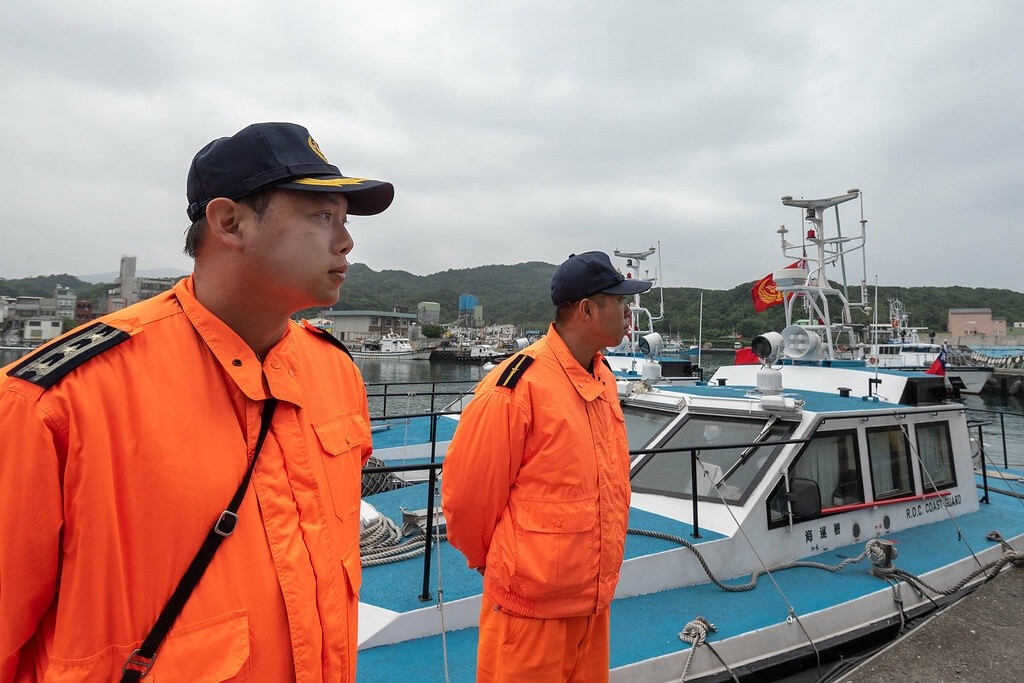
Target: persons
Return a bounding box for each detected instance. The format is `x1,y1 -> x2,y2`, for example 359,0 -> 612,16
441,251 -> 653,681
0,122 -> 396,683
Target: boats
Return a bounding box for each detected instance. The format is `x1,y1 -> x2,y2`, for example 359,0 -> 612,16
308,183 -> 1023,683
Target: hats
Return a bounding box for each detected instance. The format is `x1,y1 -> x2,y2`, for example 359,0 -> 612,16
549,250 -> 652,304
186,122 -> 393,218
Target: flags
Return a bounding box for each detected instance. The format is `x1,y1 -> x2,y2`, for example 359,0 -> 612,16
926,344 -> 945,376
751,259 -> 805,314
